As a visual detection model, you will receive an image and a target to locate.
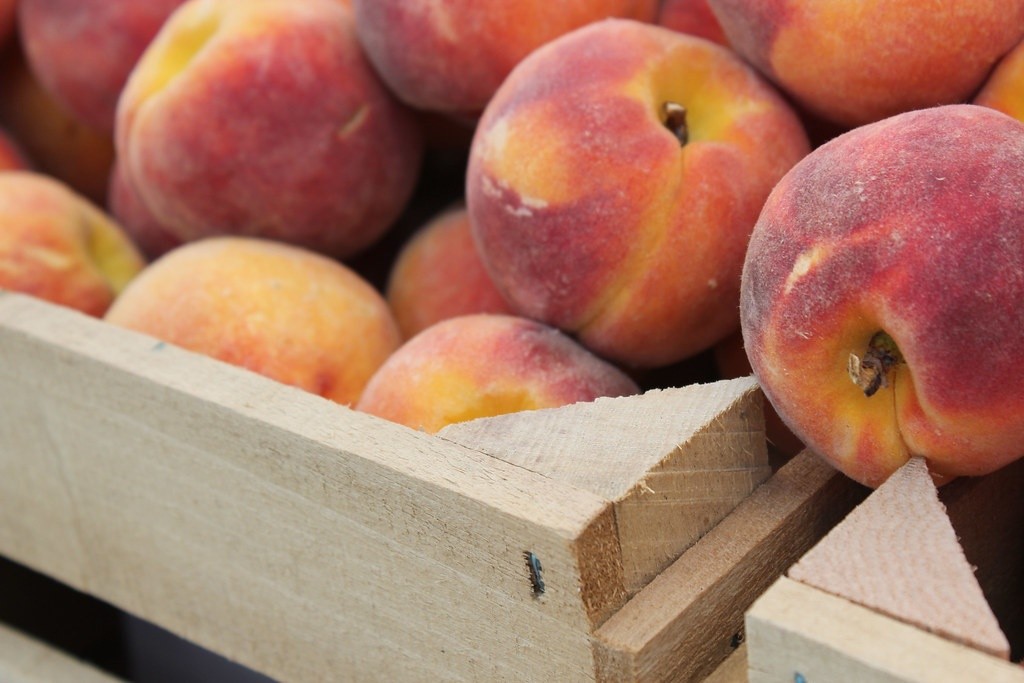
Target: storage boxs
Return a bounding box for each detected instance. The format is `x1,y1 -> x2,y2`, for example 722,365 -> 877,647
0,282 -> 1024,683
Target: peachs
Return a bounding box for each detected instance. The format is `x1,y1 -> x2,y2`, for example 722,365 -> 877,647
0,0 -> 1024,488
